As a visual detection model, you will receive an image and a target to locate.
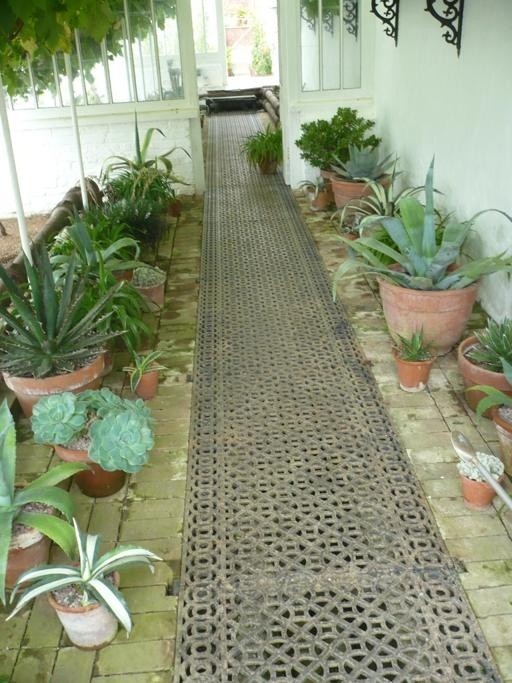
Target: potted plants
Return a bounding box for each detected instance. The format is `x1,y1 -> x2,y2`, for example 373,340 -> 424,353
2,127 -> 191,418
29,387 -> 158,498
6,518 -> 162,647
122,350 -> 171,399
1,399 -> 96,603
293,103 -> 509,513
240,124 -> 284,176
